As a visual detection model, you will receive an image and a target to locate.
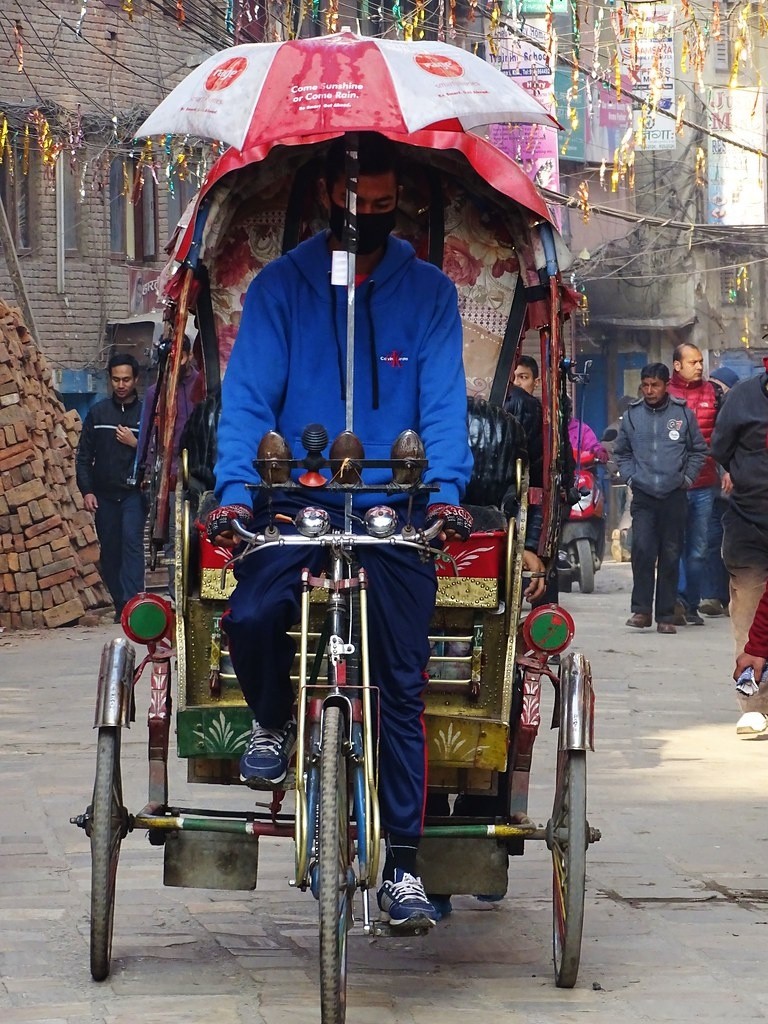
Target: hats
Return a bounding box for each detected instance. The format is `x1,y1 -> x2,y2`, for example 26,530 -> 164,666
708,367 -> 740,397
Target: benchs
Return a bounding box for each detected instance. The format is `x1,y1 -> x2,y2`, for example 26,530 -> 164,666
177,383 -> 524,607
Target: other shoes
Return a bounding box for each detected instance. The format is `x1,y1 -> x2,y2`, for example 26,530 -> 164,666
113,610 -> 121,624
682,612 -> 704,625
697,599 -> 723,615
725,602 -> 730,617
657,622 -> 676,633
626,612 -> 652,628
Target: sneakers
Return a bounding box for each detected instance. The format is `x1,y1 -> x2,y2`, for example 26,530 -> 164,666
376,868 -> 437,930
239,713 -> 297,790
736,711 -> 768,733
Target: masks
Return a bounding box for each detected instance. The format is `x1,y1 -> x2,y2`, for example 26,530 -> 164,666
328,199 -> 395,258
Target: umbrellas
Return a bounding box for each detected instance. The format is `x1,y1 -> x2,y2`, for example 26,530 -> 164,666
131,26 -> 565,141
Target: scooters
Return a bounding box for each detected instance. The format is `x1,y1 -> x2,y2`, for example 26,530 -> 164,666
551,427 -> 619,595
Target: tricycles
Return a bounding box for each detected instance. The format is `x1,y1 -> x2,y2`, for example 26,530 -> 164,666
68,25 -> 602,1024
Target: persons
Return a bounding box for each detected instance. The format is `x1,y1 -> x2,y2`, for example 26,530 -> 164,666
74,355 -> 148,622
731,580 -> 768,688
709,373 -> 768,736
597,383 -> 645,561
511,358 -> 542,395
564,394 -> 609,465
664,342 -> 724,623
202,141 -> 475,929
612,362 -> 708,634
133,331 -> 202,611
502,383 -> 548,602
699,366 -> 739,617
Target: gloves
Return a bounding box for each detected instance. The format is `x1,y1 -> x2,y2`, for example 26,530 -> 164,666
595,447 -> 609,464
